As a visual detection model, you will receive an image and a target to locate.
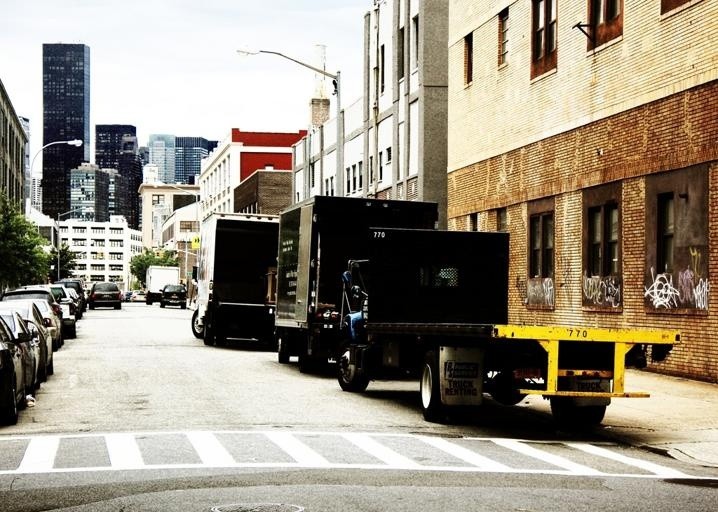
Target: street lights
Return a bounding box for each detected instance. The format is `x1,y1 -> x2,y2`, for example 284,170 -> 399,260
172,227 -> 190,289
25,139 -> 84,224
235,46 -> 347,198
55,206 -> 88,282
156,180 -> 201,284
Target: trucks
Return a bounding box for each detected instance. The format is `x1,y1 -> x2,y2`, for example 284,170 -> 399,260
264,193 -> 444,376
191,209 -> 283,351
331,222 -> 684,436
143,265 -> 183,306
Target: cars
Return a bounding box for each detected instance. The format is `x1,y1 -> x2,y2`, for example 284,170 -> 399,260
1,276 -> 92,429
122,290 -> 148,303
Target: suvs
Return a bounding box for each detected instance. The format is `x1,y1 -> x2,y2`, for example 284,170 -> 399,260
87,282 -> 123,312
160,284 -> 189,311
